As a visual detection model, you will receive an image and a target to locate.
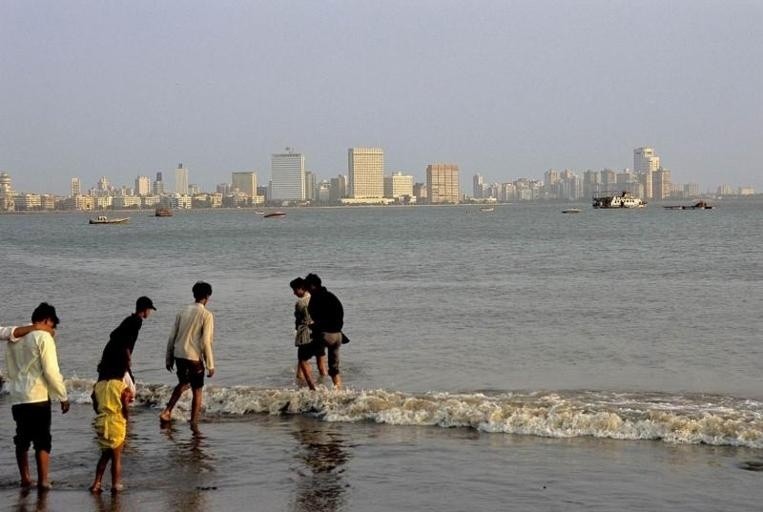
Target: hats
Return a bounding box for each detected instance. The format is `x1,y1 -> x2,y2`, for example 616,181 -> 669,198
136,296 -> 156,311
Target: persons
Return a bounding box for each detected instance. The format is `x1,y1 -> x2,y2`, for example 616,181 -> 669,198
89,355 -> 131,494
157,281 -> 217,435
5,302 -> 72,491
96,295 -> 156,396
0,322 -> 54,344
287,277 -> 328,380
295,273 -> 350,391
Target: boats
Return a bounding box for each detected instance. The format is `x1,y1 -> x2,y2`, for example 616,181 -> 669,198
479,205 -> 497,212
664,200 -> 715,211
253,210 -> 264,214
592,189 -> 649,210
155,208 -> 175,218
262,210 -> 287,218
561,207 -> 581,212
88,215 -> 129,223
